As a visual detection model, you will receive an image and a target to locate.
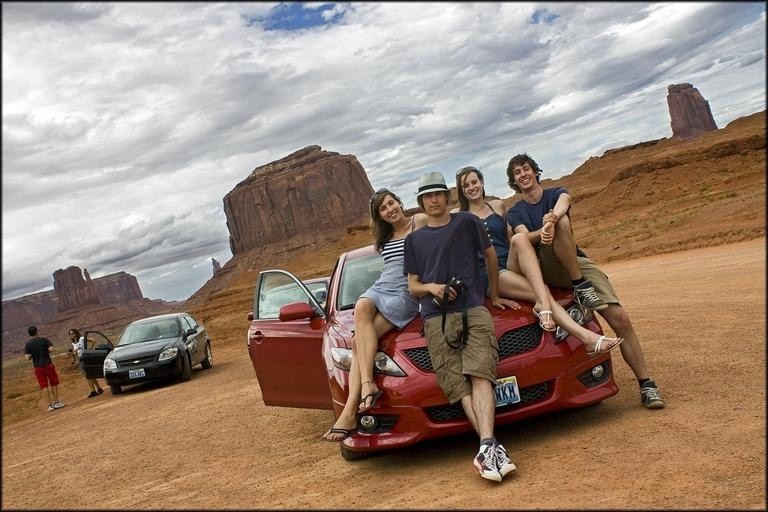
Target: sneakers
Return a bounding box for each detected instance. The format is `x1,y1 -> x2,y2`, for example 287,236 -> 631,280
55,402 -> 66,408
87,390 -> 97,399
494,444 -> 517,476
47,405 -> 54,411
97,387 -> 104,394
571,280 -> 609,310
639,381 -> 666,409
473,442 -> 502,482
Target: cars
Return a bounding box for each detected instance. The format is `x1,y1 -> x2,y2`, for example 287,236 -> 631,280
78,312 -> 217,396
246,276 -> 334,359
246,240 -> 623,462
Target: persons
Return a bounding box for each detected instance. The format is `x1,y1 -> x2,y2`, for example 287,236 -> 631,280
322,187 -> 429,442
69,329 -> 104,398
505,152 -> 664,409
449,167 -> 625,358
403,172 -> 521,482
24,326 -> 67,411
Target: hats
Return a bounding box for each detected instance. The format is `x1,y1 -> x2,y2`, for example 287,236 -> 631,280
416,171 -> 450,198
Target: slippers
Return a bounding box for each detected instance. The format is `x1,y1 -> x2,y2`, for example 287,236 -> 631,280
587,336 -> 624,358
357,389 -> 383,413
532,306 -> 556,331
322,426 -> 358,441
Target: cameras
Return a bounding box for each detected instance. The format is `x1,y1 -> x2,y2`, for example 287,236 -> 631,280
430,271 -> 469,310
